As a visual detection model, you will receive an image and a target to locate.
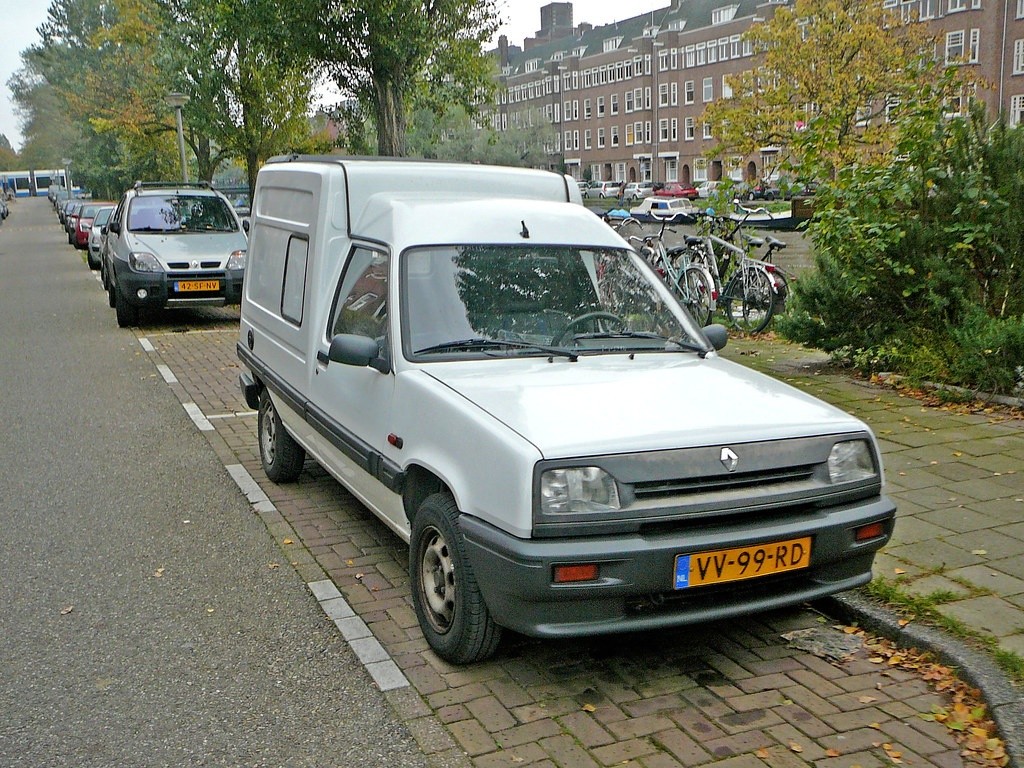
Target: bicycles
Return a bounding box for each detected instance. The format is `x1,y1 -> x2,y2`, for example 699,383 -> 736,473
594,198 -> 794,334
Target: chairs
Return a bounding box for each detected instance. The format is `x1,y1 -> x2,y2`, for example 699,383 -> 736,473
495,274 -> 575,347
191,203 -> 219,229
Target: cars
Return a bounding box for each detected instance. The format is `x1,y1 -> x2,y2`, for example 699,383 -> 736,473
585,181 -> 620,199
576,182 -> 590,197
724,182 -> 768,201
82,207 -> 115,270
695,181 -> 735,198
48,185 -> 82,244
616,183 -> 656,201
72,202 -> 117,249
654,182 -> 699,201
99,205 -> 181,291
0,200 -> 9,220
762,181 -> 821,201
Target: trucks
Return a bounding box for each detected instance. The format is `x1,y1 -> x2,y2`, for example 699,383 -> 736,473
237,154 -> 897,666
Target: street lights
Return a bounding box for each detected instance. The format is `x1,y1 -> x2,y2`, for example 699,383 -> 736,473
61,158 -> 72,200
165,93 -> 190,188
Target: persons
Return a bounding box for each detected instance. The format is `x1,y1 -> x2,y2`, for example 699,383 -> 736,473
618,181 -> 627,207
653,182 -> 665,192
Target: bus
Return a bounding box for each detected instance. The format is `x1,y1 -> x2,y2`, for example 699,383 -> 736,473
0,169 -> 92,197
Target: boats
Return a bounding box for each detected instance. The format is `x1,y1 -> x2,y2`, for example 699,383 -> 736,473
722,196 -> 842,232
630,196 -> 702,225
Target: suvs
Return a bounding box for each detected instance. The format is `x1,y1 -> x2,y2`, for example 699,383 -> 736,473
106,181 -> 251,327
